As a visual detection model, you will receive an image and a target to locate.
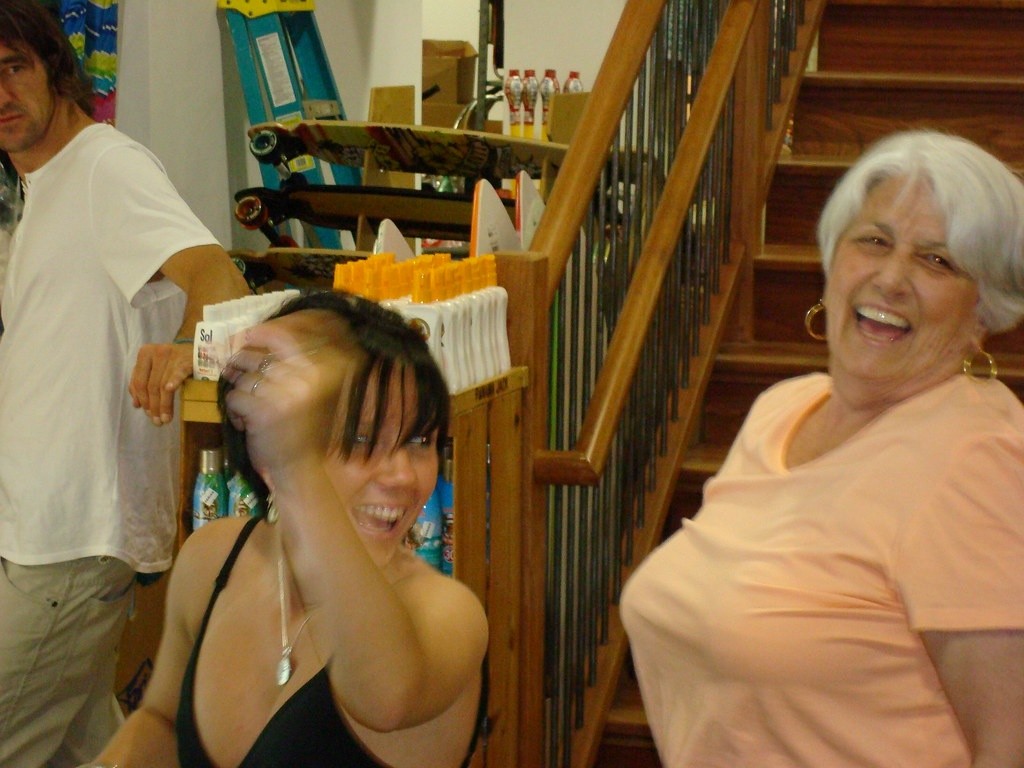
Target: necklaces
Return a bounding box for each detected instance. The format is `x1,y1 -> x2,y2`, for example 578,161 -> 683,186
274,526 -> 311,686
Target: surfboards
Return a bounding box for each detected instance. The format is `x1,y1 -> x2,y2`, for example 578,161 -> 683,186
470,178 -> 523,258
515,168 -> 546,249
373,217 -> 419,261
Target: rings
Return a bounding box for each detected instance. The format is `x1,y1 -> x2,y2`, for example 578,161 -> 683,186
255,352 -> 273,371
250,377 -> 265,393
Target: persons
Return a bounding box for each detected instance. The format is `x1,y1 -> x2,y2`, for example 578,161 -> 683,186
619,130 -> 1024,768
0,0 -> 253,768
75,289 -> 488,768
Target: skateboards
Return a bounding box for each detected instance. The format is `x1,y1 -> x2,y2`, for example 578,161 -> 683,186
242,117 -> 651,184
228,247 -> 474,291
233,184 -> 642,252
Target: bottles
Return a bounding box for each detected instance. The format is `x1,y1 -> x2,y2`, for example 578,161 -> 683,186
539,70 -> 560,124
404,460 -> 453,575
506,70 -> 524,124
192,446 -> 264,533
523,70 -> 539,123
563,71 -> 583,93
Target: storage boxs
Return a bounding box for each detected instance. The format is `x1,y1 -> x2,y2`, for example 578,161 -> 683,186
422,39 -> 479,132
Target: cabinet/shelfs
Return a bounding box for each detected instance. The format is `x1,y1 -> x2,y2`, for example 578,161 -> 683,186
177,366 -> 530,768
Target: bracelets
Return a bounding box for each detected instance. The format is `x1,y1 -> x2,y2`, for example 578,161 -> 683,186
175,338 -> 194,344
